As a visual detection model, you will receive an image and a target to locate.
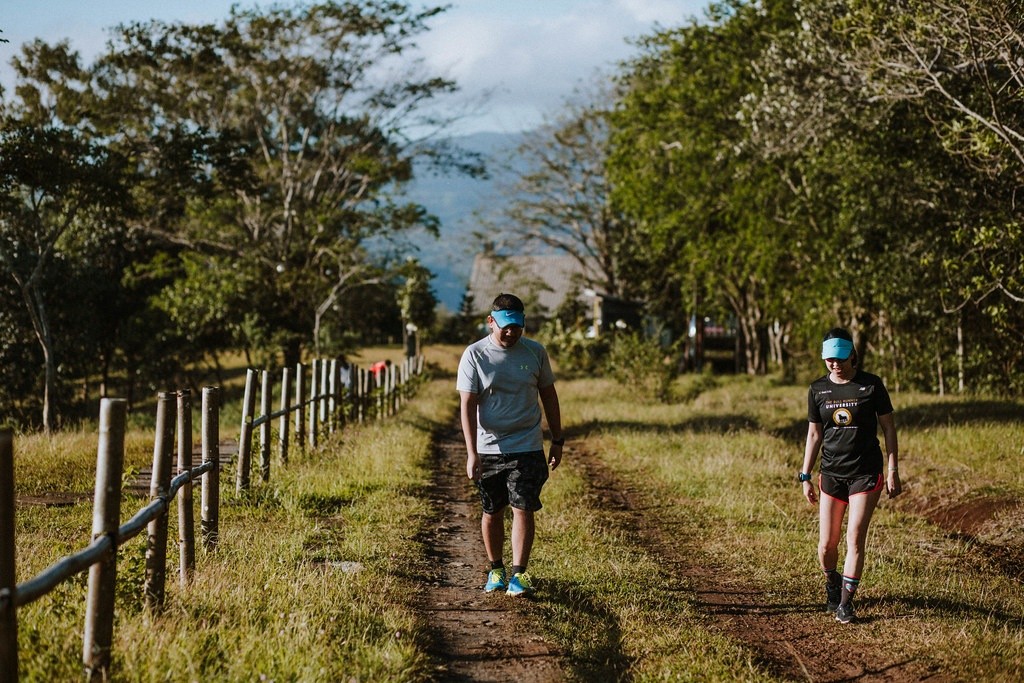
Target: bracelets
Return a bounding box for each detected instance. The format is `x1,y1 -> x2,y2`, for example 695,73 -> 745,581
888,466 -> 898,470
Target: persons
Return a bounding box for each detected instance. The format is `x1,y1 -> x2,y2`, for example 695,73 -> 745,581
455,293 -> 564,596
798,328 -> 903,626
405,322 -> 418,359
337,354 -> 350,388
369,359 -> 392,379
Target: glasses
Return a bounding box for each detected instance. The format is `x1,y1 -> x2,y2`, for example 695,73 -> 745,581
826,350 -> 854,363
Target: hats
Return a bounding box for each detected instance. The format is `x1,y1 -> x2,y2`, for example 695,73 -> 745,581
821,337 -> 854,359
491,310 -> 525,329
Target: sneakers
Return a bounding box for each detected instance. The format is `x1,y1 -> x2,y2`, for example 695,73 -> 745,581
825,574 -> 842,611
836,604 -> 856,623
505,572 -> 536,597
485,570 -> 506,592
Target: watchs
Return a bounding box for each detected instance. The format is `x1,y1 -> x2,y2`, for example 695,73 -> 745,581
798,472 -> 812,482
552,436 -> 565,446
